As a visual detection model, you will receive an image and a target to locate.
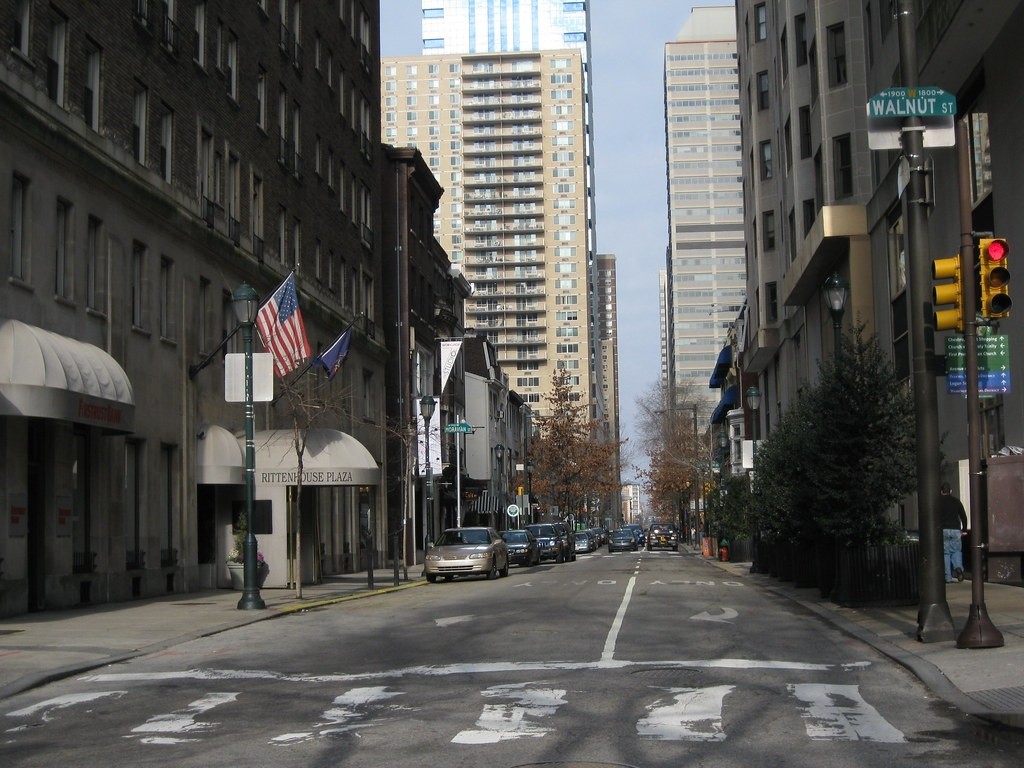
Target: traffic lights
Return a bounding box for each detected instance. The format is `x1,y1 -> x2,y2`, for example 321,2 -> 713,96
705,483 -> 711,496
977,238 -> 1013,319
930,254 -> 966,333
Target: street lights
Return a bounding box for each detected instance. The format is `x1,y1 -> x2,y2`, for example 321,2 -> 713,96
494,443 -> 506,533
716,429 -> 732,505
232,279 -> 267,610
527,462 -> 537,524
653,404 -> 702,552
686,479 -> 693,546
419,394 -> 440,578
746,385 -> 762,495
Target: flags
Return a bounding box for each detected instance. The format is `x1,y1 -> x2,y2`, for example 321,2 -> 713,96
250,270 -> 312,379
312,323 -> 352,381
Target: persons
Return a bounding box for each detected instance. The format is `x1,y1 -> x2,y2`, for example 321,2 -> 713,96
690,525 -> 696,546
940,481 -> 968,584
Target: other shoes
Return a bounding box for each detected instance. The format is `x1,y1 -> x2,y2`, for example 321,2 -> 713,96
956,567 -> 964,582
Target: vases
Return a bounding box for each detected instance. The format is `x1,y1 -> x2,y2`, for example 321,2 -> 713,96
227,562 -> 264,591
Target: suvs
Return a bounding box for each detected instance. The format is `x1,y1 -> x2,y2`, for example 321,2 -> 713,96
524,523 -> 564,565
554,522 -> 577,563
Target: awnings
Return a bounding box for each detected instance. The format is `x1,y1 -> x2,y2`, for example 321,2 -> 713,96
710,386 -> 740,425
708,345 -> 733,389
198,422 -> 381,488
0,315 -> 137,439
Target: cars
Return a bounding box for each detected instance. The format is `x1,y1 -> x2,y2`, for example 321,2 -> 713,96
498,530 -> 541,567
424,527 -> 509,583
573,523 -> 679,554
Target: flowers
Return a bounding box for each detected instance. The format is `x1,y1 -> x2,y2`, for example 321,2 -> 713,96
225,512 -> 264,564
